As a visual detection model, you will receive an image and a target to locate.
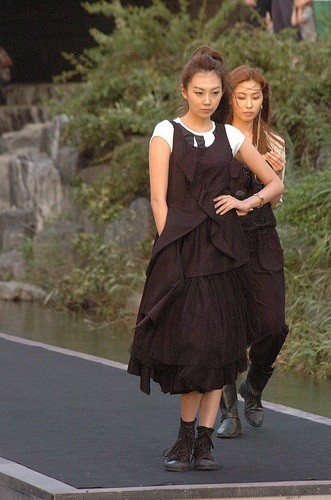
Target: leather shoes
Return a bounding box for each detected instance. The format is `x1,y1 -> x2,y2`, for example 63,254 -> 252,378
162,424 -> 196,473
190,430 -> 219,470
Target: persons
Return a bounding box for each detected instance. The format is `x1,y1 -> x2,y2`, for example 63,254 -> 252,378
127,46 -> 283,470
216,65 -> 288,437
246,0 -> 320,43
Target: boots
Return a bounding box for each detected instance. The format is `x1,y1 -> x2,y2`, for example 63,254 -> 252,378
238,363 -> 275,428
215,382 -> 243,439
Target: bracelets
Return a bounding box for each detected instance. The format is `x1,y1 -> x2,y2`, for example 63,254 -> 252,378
254,193 -> 264,208
